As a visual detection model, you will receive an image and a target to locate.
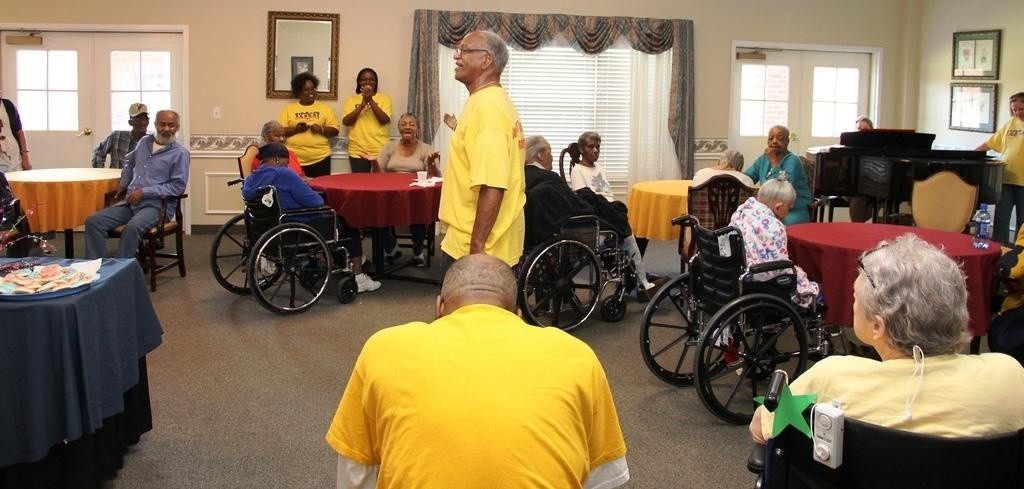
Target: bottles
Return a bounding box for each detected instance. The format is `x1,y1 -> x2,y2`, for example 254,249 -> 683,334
972,202 -> 991,250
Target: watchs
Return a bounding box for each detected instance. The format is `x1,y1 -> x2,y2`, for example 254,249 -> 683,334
428,160 -> 437,166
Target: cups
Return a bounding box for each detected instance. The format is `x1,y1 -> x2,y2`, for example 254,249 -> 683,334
417,171 -> 428,184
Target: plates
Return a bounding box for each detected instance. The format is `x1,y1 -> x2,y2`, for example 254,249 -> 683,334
0,265 -> 101,300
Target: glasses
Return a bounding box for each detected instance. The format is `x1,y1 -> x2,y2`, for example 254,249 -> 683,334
857,241 -> 890,288
455,46 -> 488,57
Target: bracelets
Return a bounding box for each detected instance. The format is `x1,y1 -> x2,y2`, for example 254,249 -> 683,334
20,150 -> 30,153
321,127 -> 325,134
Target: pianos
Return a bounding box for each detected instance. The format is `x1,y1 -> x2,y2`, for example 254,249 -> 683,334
805,128 -> 1006,224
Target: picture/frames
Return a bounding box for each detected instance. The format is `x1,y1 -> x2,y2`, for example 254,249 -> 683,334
949,83 -> 998,133
291,55 -> 314,82
951,29 -> 1002,80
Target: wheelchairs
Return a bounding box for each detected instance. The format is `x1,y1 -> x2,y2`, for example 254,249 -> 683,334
210,178 -> 359,315
746,369 -> 1024,489
516,214 -> 657,332
639,215 -> 849,426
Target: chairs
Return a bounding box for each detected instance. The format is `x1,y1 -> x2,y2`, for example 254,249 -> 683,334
558,143 -> 581,193
237,145 -> 260,186
797,156 -> 823,223
910,169 -> 981,235
688,174 -> 759,266
391,155 -> 441,268
104,189 -> 188,292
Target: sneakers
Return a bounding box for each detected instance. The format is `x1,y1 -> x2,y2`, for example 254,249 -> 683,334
344,255 -> 367,274
638,275 -> 669,303
356,273 -> 381,294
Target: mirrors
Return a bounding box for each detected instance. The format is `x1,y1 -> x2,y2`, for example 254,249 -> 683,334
266,11 -> 341,101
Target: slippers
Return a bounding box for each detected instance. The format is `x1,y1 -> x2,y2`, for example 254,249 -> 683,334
384,251 -> 402,266
411,256 -> 424,268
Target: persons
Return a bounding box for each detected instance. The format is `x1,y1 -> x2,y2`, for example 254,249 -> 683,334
91,103 -> 149,168
324,253 -> 630,489
341,67 -> 392,172
749,233 -> 1024,445
524,136 -> 669,303
973,91 -> 1024,242
241,143 -> 380,293
85,110 -> 190,258
856,114 -> 873,133
438,31 -> 527,282
378,113 -> 442,176
0,87 -> 32,171
729,170 -> 820,309
251,121 -> 314,183
692,149 -> 753,188
278,73 -> 340,178
743,126 -> 813,224
570,131 -> 615,205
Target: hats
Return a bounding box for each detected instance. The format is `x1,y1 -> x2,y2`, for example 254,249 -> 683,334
256,142 -> 289,159
129,103 -> 150,118
